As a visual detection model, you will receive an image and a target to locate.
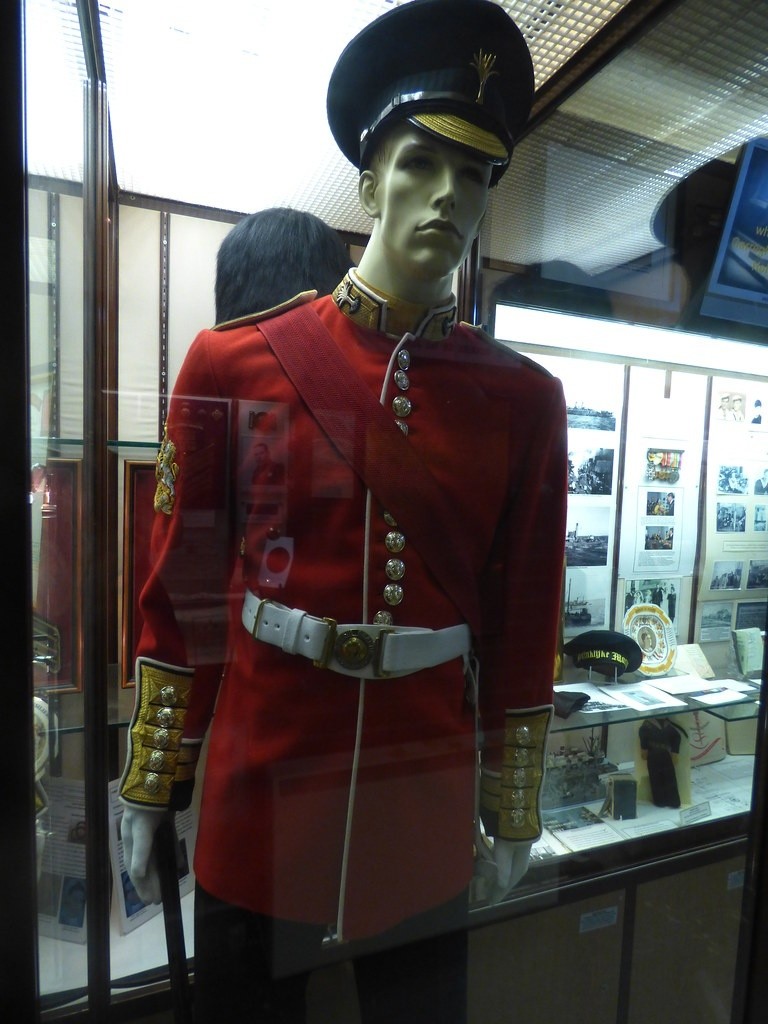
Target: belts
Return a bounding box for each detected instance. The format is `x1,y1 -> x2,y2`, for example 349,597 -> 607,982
240,589 -> 471,676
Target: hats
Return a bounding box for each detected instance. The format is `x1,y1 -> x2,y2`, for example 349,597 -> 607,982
328,0 -> 536,183
563,629 -> 642,675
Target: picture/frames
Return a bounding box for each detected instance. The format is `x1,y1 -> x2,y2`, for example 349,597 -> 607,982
120,457 -> 157,692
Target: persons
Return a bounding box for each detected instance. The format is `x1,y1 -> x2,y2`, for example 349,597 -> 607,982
116,0 -> 568,1023
625,394 -> 768,622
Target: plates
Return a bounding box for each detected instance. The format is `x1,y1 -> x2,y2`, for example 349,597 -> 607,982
33,697 -> 58,782
622,603 -> 677,676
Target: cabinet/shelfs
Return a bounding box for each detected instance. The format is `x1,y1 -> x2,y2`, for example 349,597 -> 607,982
457,661 -> 768,1024
